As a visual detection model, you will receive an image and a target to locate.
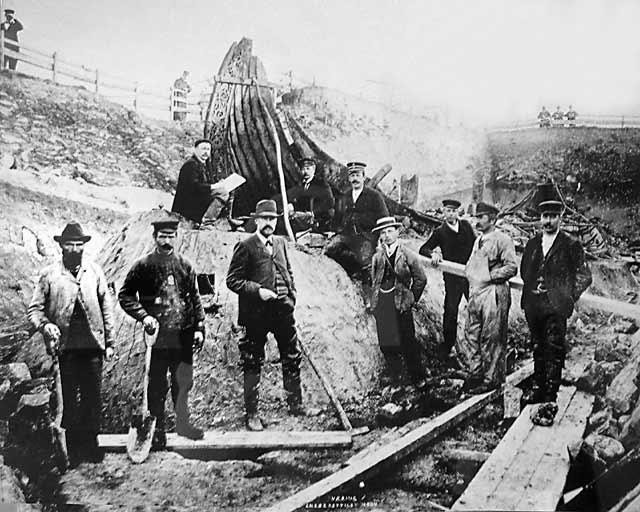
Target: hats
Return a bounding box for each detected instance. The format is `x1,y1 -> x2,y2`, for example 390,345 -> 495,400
151,221 -> 179,233
54,222 -> 91,244
250,199 -> 282,217
539,201 -> 563,215
442,199 -> 461,209
298,158 -> 316,167
371,217 -> 403,233
474,202 -> 500,216
195,139 -> 212,148
347,162 -> 367,172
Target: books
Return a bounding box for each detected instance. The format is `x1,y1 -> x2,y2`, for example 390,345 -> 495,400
217,171 -> 247,194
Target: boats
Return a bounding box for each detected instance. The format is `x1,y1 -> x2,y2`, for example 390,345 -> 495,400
199,36 -> 483,248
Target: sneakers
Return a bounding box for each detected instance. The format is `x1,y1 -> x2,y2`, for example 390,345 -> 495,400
248,417 -> 263,431
293,406 -> 323,417
177,422 -> 204,439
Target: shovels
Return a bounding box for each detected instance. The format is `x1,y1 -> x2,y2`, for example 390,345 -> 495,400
47,331 -> 71,475
126,322 -> 159,464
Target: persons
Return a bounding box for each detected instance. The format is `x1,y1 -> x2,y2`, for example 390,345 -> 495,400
521,200 -> 595,407
366,216 -> 428,392
325,162 -> 390,286
0,8 -> 24,70
171,138 -> 231,231
540,104 -> 581,130
119,218 -> 205,449
274,158 -> 336,233
27,220 -> 116,469
462,201 -> 517,393
172,71 -> 191,120
227,198 -> 317,431
419,199 -> 476,356
